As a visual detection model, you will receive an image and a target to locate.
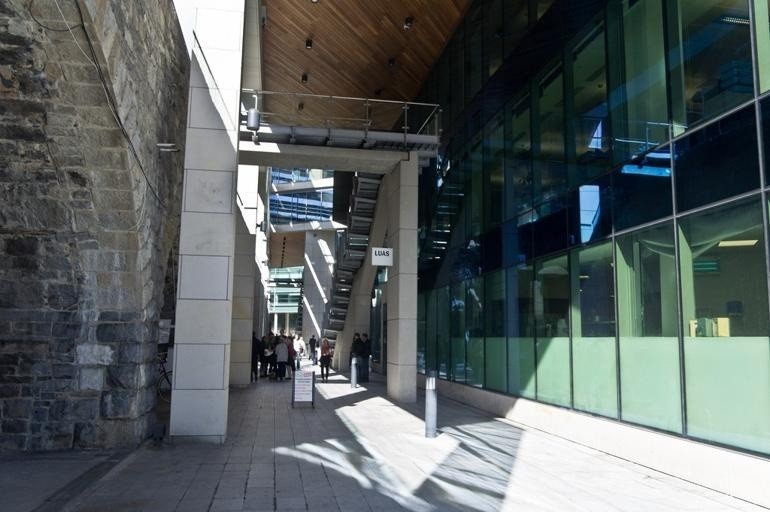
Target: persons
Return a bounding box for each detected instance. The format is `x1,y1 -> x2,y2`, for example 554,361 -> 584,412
250,329 -> 373,388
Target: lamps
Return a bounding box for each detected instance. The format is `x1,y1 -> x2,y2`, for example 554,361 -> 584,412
310,1 -> 321,5
300,72 -> 309,84
402,16 -> 414,32
305,39 -> 313,50
297,101 -> 305,114
